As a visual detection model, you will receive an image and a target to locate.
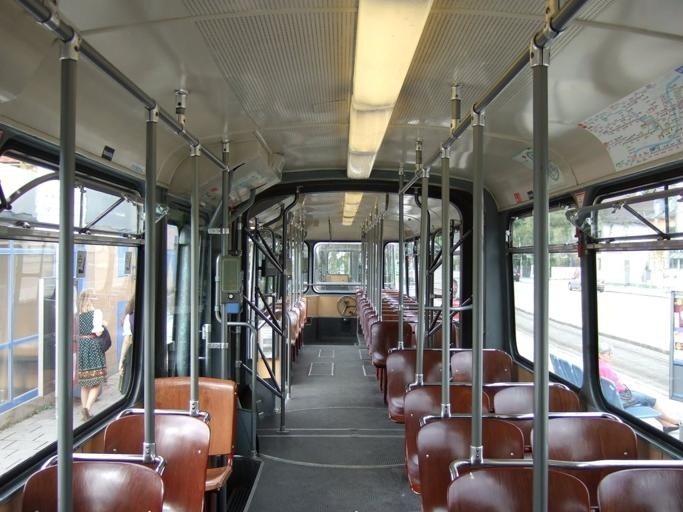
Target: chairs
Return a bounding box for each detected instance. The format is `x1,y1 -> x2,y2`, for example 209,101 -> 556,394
357,290 -> 461,409
548,352 -> 666,420
387,347 -> 683,511
20,376 -> 239,512
256,299 -> 308,360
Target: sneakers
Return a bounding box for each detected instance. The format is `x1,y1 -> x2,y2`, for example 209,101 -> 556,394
81,407 -> 92,420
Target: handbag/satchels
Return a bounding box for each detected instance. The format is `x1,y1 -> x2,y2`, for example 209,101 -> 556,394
96,324 -> 111,352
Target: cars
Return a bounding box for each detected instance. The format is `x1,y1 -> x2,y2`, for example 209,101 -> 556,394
568,276 -> 605,293
513,269 -> 521,281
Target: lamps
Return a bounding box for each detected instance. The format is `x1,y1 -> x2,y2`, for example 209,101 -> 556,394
346,1 -> 438,180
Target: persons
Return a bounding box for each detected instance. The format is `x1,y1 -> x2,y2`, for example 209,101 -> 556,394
73,287 -> 111,421
595,341 -> 681,434
452,279 -> 460,323
117,291 -> 135,396
